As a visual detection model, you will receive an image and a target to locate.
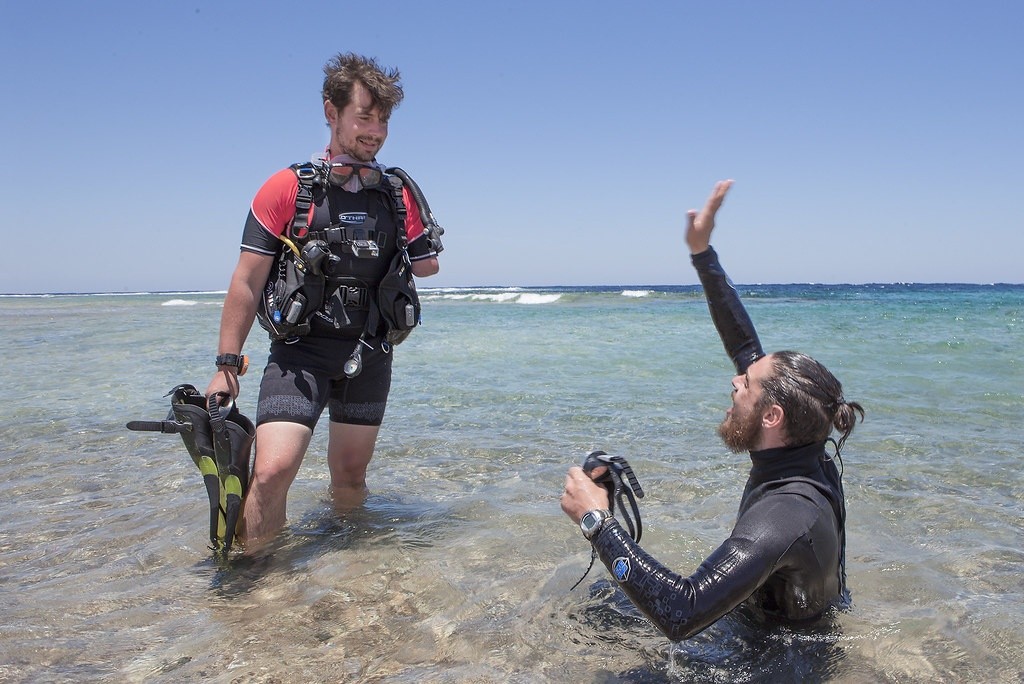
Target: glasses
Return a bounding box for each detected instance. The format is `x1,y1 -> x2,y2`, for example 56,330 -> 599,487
327,162 -> 383,189
583,451 -> 645,544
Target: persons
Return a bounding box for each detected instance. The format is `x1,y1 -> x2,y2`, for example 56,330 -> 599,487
559,180 -> 863,642
206,52 -> 443,540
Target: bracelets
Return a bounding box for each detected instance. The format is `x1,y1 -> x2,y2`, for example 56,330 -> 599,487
215,354 -> 248,376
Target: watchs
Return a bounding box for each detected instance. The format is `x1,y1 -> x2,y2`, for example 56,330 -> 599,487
580,509 -> 611,540
